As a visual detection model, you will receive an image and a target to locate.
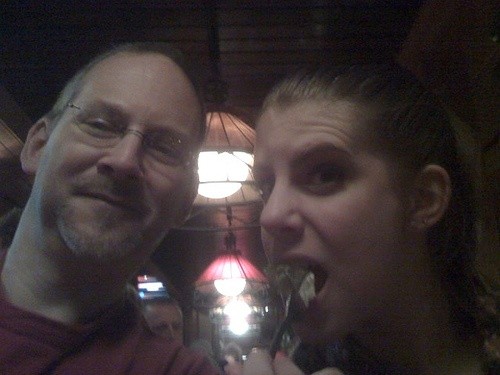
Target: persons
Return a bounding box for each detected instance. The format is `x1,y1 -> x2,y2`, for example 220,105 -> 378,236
0,39 -> 223,375
140,296 -> 222,375
244,55 -> 499,375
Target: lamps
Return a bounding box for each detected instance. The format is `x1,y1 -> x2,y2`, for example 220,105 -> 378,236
171,0 -> 271,340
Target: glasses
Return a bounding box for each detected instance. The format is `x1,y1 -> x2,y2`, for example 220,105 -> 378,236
50,103 -> 198,171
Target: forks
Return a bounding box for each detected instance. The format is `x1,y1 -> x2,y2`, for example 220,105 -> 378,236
269,286 -> 300,360
